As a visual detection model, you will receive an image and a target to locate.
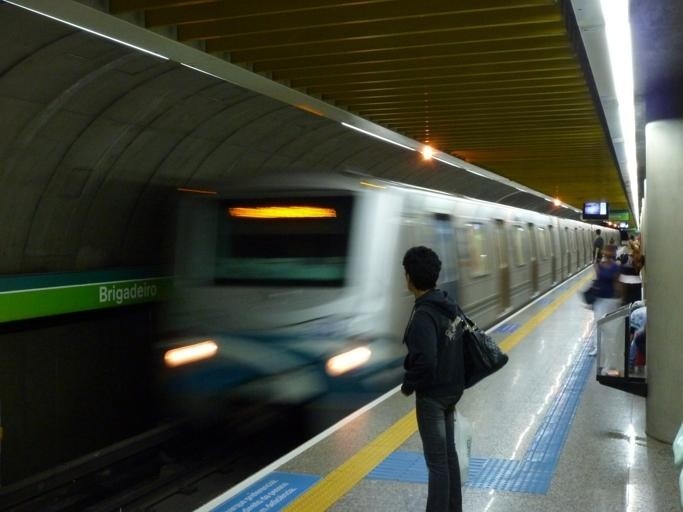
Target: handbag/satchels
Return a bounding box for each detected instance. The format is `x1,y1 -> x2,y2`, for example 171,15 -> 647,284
460,316 -> 510,390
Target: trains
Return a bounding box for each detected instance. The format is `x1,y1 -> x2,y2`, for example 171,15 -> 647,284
153,171 -> 621,447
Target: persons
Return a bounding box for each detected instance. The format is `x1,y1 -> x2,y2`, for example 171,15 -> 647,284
403,245 -> 467,512
584,229 -> 647,374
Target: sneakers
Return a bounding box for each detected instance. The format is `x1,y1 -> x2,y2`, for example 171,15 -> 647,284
588,347 -> 597,357
